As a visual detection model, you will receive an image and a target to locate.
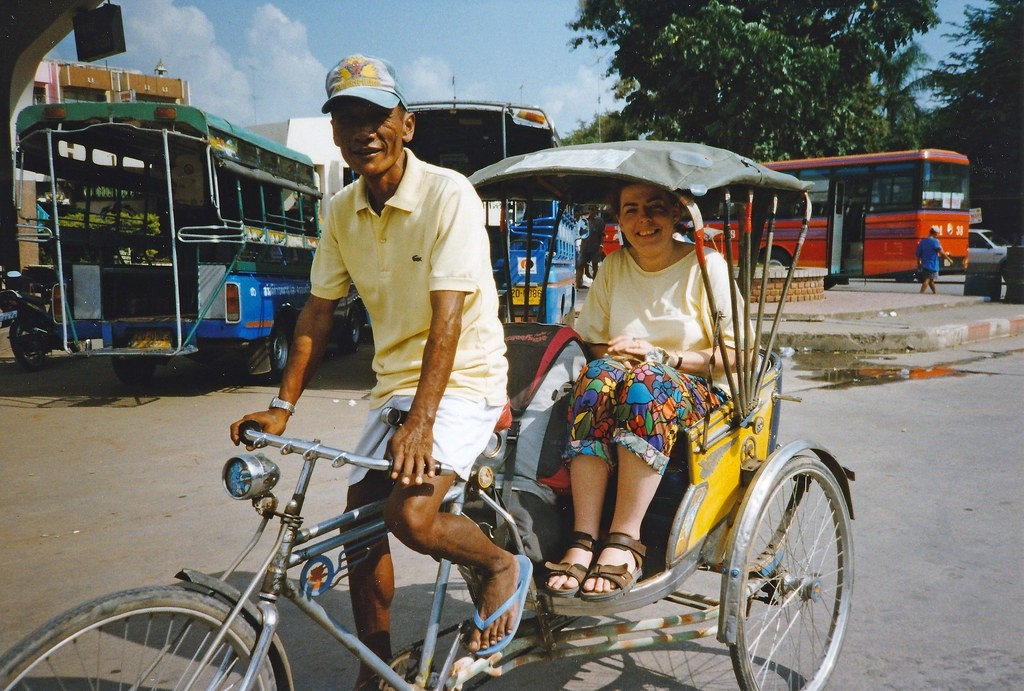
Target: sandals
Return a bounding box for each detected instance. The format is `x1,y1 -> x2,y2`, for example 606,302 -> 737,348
580,530 -> 648,602
545,531 -> 600,597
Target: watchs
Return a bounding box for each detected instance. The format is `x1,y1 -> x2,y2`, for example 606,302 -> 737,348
268,397 -> 296,416
673,349 -> 683,370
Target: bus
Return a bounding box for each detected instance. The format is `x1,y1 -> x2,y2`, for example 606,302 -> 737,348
405,98 -> 590,328
692,147 -> 972,283
12,101 -> 321,387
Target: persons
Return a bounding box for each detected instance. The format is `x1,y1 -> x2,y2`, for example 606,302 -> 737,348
916,225 -> 954,294
227,54 -> 533,691
542,176 -> 763,606
579,204 -> 605,285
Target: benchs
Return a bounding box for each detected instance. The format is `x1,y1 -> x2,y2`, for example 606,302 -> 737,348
196,261 -> 307,319
72,263 -> 174,321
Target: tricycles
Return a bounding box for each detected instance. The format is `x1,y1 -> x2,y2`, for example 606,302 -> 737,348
0,136 -> 858,690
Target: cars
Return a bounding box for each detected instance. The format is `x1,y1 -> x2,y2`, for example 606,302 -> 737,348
968,228 -> 1024,275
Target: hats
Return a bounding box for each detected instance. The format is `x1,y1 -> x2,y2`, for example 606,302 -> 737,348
321,53 -> 408,115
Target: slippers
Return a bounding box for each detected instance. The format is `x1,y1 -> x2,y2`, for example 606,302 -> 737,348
480,554 -> 534,655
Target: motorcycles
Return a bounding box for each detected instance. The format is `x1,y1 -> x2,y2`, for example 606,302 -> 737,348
0,269 -> 94,372
